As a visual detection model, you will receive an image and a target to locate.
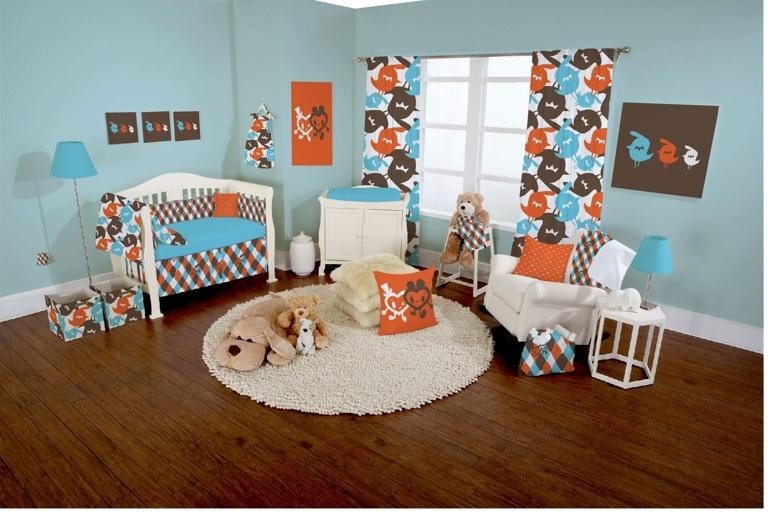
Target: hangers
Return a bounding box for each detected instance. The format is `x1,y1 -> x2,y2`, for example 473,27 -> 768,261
251,97 -> 276,122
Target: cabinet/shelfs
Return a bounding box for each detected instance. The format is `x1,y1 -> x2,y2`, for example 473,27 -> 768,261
317,186 -> 410,277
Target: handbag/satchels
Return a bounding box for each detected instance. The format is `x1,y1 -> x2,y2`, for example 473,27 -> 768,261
519,323 -> 577,377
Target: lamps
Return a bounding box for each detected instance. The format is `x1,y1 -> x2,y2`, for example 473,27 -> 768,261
630,234 -> 673,311
50,141 -> 98,288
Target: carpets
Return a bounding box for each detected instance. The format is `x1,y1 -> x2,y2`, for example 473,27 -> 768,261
203,286 -> 495,417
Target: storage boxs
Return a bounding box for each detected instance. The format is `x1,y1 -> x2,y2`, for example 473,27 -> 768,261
90,275 -> 147,330
44,287 -> 106,342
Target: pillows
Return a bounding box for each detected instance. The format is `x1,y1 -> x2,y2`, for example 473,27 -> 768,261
329,253 -> 440,336
212,193 -> 241,218
326,188 -> 402,203
512,235 -> 574,283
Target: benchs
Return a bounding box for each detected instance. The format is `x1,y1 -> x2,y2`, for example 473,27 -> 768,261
96,171 -> 279,320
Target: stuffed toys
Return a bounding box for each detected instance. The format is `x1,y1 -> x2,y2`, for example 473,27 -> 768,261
279,294 -> 325,348
438,193 -> 489,269
295,318 -> 320,356
214,291 -> 293,372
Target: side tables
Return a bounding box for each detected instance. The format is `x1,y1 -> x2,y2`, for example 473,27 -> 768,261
588,298 -> 666,389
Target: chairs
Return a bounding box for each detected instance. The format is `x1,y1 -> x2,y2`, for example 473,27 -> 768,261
435,227 -> 495,297
482,229 -> 638,344
